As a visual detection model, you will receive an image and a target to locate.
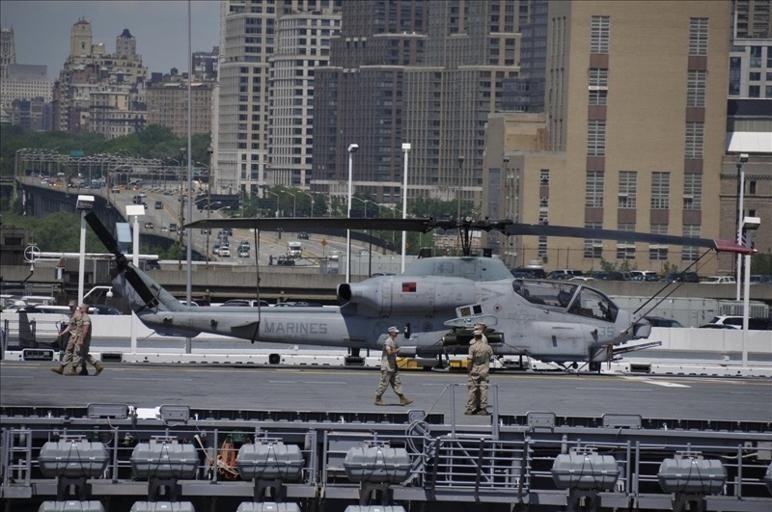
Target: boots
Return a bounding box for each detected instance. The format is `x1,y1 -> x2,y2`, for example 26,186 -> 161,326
375,395 -> 384,405
399,394 -> 413,405
51,361 -> 104,375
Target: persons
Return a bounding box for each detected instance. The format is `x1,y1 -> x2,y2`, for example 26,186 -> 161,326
375,327 -> 414,406
470,325 -> 488,414
464,332 -> 494,415
64,305 -> 103,375
51,301 -> 89,375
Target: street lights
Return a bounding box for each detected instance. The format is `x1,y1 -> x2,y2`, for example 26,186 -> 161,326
345,142 -> 359,284
735,150 -> 750,304
73,193 -> 94,317
123,201 -> 145,352
739,216 -> 762,370
399,142 -> 412,275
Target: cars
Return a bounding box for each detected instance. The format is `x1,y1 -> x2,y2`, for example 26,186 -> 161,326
512,265 -> 772,284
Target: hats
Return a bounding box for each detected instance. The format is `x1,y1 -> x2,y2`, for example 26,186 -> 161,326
388,327 -> 399,332
473,326 -> 482,335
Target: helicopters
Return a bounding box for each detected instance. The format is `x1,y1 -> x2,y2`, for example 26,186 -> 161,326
82,216 -> 762,377
